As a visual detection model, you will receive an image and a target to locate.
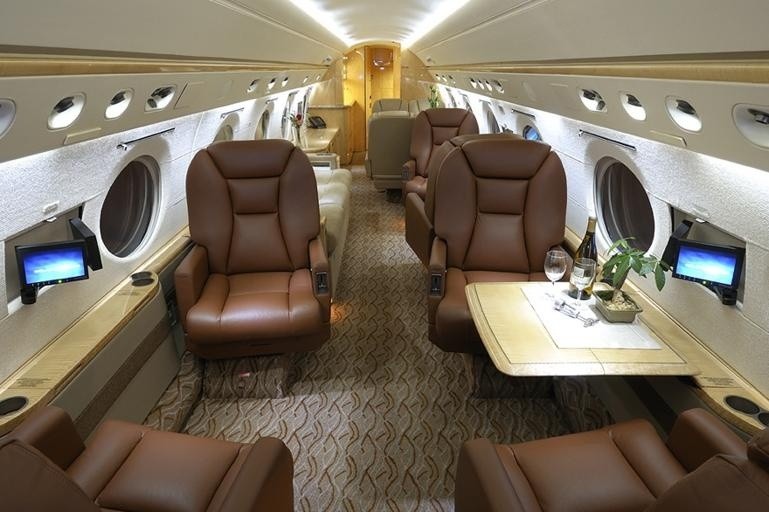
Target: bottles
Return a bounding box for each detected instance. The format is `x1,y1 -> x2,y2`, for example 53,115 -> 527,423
569,217 -> 598,300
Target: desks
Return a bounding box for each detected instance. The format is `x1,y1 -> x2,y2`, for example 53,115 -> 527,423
465,281 -> 689,377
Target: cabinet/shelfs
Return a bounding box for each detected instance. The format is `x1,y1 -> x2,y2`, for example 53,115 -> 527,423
300,99 -> 357,169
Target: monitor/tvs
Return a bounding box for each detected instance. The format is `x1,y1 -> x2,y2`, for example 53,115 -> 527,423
310,115 -> 326,127
657,220 -> 692,272
70,217 -> 104,270
14,239 -> 89,291
670,238 -> 745,289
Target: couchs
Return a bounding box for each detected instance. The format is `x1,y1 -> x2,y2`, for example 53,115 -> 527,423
364,98 -> 527,266
309,169 -> 353,299
454,406 -> 769,510
2,406 -> 294,512
175,140 -> 332,358
428,138 -> 568,351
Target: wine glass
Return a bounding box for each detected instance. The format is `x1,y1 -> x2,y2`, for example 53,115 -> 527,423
571,258 -> 597,309
544,250 -> 568,297
